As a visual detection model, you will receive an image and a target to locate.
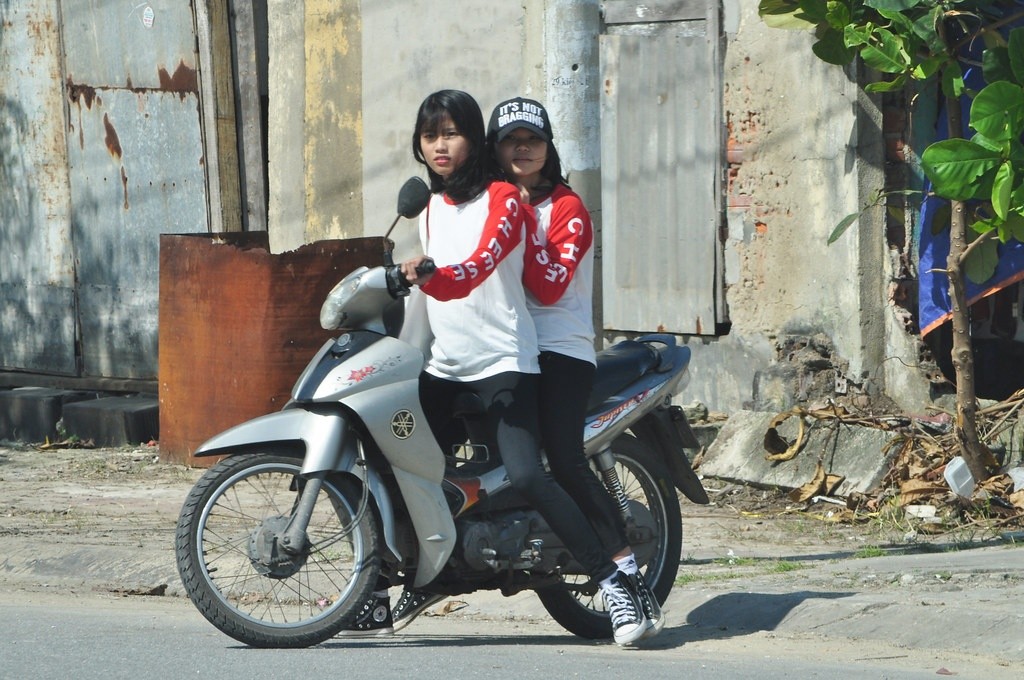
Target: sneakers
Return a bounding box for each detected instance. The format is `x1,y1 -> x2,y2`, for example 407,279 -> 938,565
600,570 -> 646,645
331,595 -> 395,639
390,587 -> 449,632
628,570 -> 664,638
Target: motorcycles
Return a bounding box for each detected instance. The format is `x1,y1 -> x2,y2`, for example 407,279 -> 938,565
175,175 -> 710,648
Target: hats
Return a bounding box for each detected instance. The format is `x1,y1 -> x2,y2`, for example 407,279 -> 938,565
486,97 -> 553,144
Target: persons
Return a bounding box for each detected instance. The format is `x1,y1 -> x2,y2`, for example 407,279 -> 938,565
333,90 -> 647,645
392,97 -> 665,639
